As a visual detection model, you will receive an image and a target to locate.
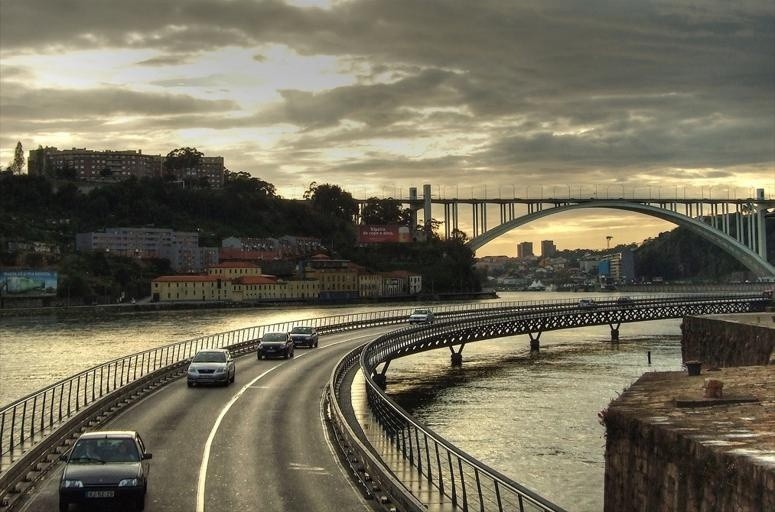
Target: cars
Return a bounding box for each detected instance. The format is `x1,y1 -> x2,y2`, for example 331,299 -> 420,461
578,299 -> 598,310
257,333 -> 294,361
185,347 -> 234,387
59,430 -> 152,512
409,309 -> 436,325
291,327 -> 319,350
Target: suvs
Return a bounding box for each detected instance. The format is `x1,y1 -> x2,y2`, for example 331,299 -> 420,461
617,297 -> 636,307
